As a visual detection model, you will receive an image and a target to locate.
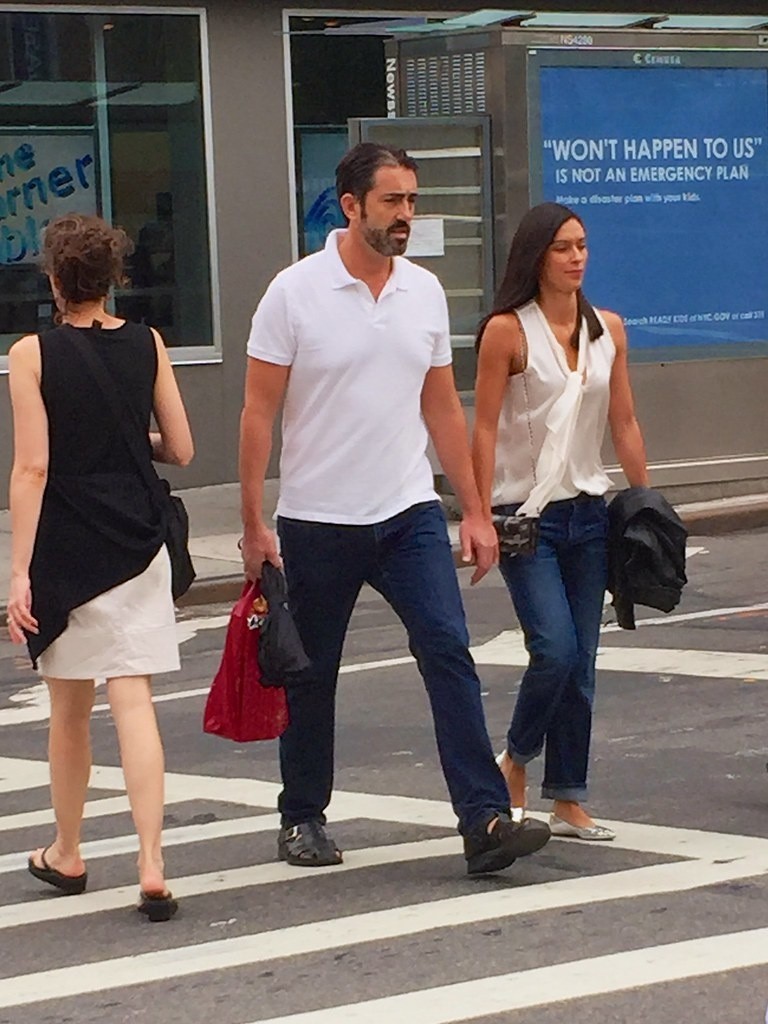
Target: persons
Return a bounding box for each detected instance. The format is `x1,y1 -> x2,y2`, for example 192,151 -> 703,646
462,200 -> 652,843
235,140 -> 554,876
2,213 -> 197,925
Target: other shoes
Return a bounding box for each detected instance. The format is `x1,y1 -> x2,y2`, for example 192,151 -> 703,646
492,752 -> 528,829
546,811 -> 617,843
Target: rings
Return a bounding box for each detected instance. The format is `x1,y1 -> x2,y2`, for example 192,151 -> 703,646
6,615 -> 15,624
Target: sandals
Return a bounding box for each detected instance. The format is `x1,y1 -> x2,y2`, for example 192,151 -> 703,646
461,812 -> 550,878
274,819 -> 346,867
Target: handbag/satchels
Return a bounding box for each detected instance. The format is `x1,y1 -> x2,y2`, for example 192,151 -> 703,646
159,481 -> 199,599
477,514 -> 541,560
203,574 -> 291,745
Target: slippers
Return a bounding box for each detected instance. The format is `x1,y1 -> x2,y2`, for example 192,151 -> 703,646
24,843 -> 87,895
136,888 -> 182,925
